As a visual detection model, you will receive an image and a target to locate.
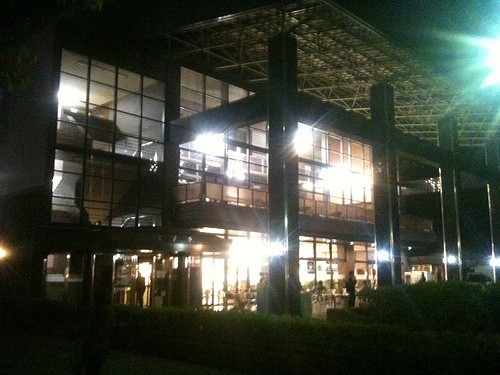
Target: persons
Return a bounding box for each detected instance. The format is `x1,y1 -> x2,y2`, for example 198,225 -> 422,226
112,244 -> 442,321
